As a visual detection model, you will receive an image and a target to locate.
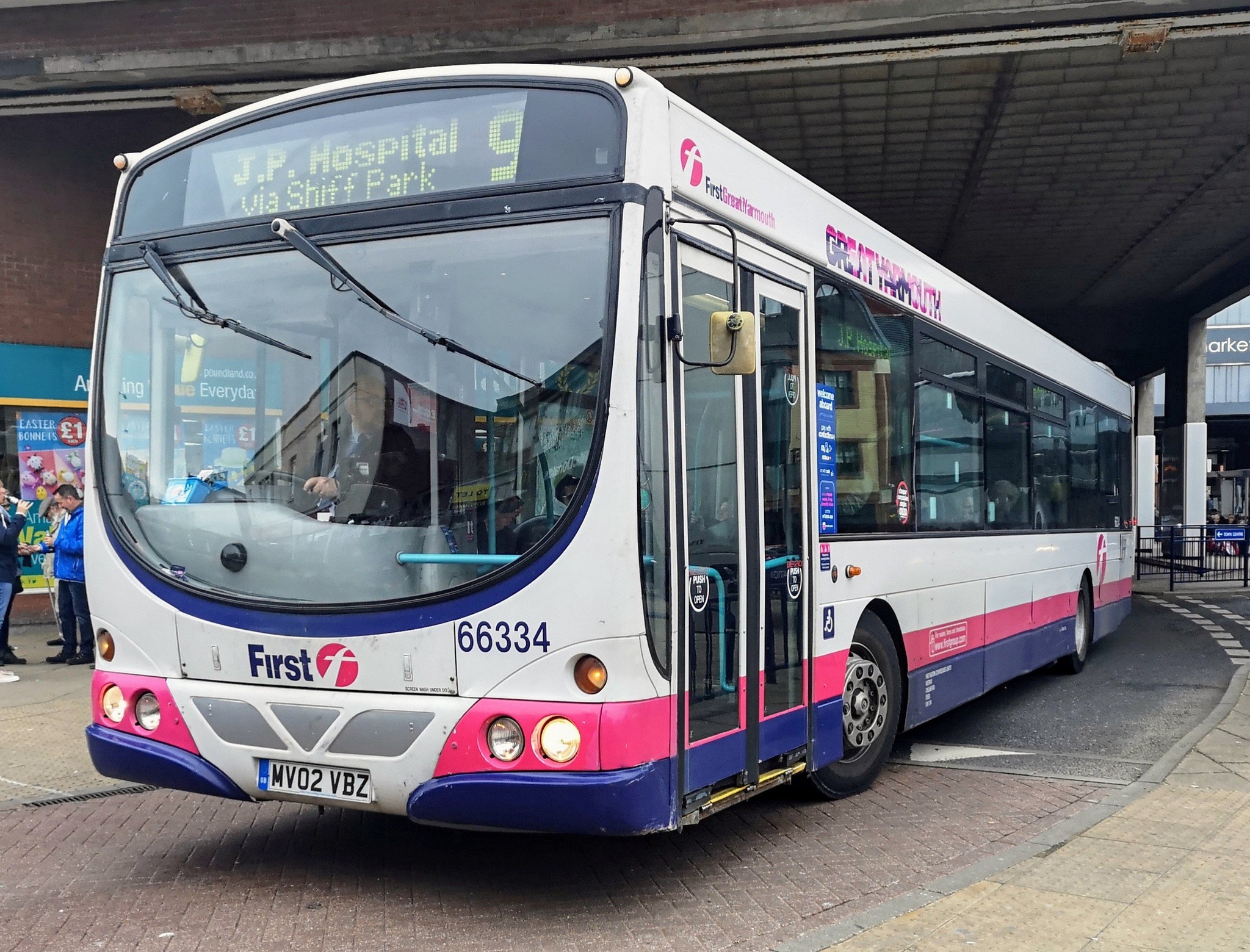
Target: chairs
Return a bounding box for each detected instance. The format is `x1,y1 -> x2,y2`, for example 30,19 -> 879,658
1053,486 -> 1115,529
399,448 -> 433,522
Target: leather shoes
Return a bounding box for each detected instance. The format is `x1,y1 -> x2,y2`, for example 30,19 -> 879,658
0,651 -> 27,666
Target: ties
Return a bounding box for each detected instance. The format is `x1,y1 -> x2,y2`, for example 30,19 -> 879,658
317,435 -> 366,513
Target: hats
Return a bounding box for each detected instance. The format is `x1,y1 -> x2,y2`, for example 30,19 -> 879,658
555,475 -> 581,503
494,501 -> 512,513
508,496 -> 524,511
37,497 -> 54,518
213,447 -> 254,470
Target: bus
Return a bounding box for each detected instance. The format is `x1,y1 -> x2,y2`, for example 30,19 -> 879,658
84,63 -> 1140,837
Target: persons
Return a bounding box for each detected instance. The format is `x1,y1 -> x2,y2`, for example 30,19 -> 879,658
289,375 -> 421,521
1206,514 -> 1250,557
24,483 -> 96,665
0,479 -> 34,666
476,496 -> 531,556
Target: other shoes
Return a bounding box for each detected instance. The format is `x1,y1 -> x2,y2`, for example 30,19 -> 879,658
0,669 -> 21,683
47,638 -> 64,645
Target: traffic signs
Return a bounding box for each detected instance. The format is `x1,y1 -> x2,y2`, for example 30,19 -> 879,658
1215,529 -> 1245,540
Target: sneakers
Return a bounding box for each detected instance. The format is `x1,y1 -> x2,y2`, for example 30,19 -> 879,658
46,648 -> 77,664
66,652 -> 95,665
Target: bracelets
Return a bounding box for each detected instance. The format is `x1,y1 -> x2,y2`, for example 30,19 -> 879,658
483,517 -> 489,533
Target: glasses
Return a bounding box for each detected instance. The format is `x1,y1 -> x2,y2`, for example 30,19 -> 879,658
353,396 -> 395,407
562,493 -> 574,501
716,508 -> 730,514
45,512 -> 49,517
